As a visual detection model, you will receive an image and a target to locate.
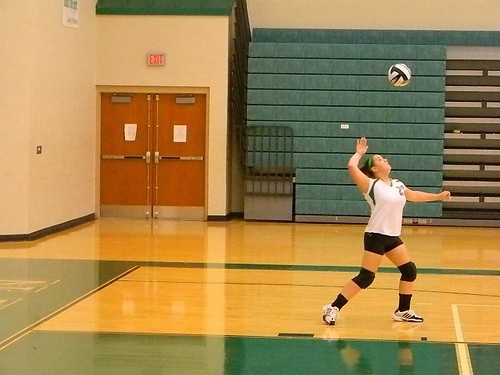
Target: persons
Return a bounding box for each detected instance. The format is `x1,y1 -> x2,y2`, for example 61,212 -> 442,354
322,137 -> 453,325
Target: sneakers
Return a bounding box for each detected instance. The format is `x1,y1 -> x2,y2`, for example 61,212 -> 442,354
322,304 -> 338,324
393,309 -> 424,322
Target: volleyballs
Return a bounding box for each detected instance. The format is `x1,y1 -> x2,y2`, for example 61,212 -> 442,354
387,63 -> 411,86
